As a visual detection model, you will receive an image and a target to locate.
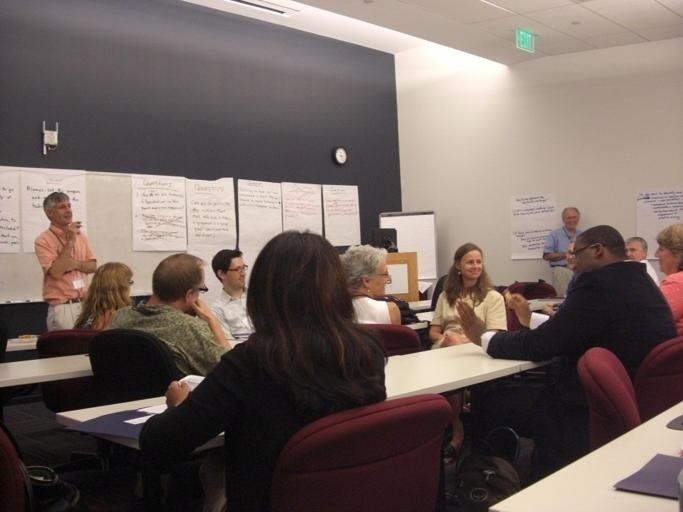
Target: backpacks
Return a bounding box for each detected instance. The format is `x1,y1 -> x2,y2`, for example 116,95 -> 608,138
458,438 -> 534,512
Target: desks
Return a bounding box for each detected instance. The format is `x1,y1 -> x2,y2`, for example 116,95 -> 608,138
488,399 -> 683,511
0,355 -> 93,422
406,311 -> 434,330
5,337 -> 38,352
408,300 -> 432,311
55,397 -> 226,512
385,340 -> 564,400
528,298 -> 566,312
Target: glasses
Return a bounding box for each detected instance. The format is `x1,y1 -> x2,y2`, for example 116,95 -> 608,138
377,271 -> 391,284
229,264 -> 247,272
568,244 -> 593,255
194,286 -> 208,294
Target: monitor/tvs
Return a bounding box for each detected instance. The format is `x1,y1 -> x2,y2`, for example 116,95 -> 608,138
372,227 -> 399,253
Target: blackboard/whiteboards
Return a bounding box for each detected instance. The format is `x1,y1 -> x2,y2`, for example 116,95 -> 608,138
0,166 -> 239,304
378,210 -> 438,281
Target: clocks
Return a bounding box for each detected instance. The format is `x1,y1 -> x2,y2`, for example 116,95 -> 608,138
333,146 -> 349,165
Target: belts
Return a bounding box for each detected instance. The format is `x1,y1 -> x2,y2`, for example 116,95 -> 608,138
65,298 -> 82,303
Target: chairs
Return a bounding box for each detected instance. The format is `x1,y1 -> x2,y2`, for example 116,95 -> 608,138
634,335 -> 683,424
36,329 -> 100,413
269,394 -> 454,512
355,323 -> 422,357
502,281 -> 557,332
0,424 -> 83,512
73,328 -> 180,512
577,348 -> 640,452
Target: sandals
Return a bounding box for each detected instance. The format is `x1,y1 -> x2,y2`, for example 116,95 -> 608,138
442,444 -> 459,464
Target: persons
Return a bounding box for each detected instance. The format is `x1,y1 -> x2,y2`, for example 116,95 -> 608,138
75,263 -> 134,329
543,207 -> 585,298
624,237 -> 660,288
195,249 -> 255,346
337,245 -> 402,326
655,224 -> 683,339
428,243 -> 508,463
35,192 -> 97,333
455,224 -> 676,467
139,230 -> 387,511
111,254 -> 231,378
